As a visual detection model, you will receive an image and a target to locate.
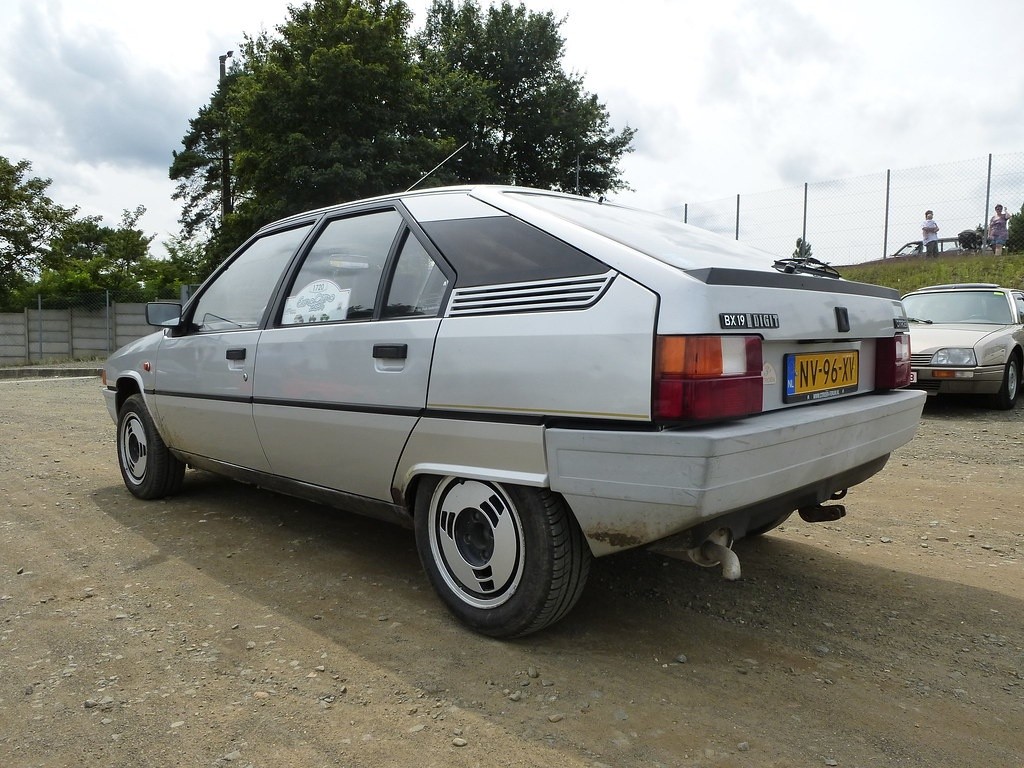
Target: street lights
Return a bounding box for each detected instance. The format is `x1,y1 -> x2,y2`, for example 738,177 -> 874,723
219,51 -> 234,216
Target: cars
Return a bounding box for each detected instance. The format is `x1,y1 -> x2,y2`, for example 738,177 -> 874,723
901,282 -> 1023,411
102,142 -> 934,640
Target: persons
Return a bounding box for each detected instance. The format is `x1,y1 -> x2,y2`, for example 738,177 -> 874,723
922,210 -> 939,260
923,214 -> 937,240
987,204 -> 1011,256
